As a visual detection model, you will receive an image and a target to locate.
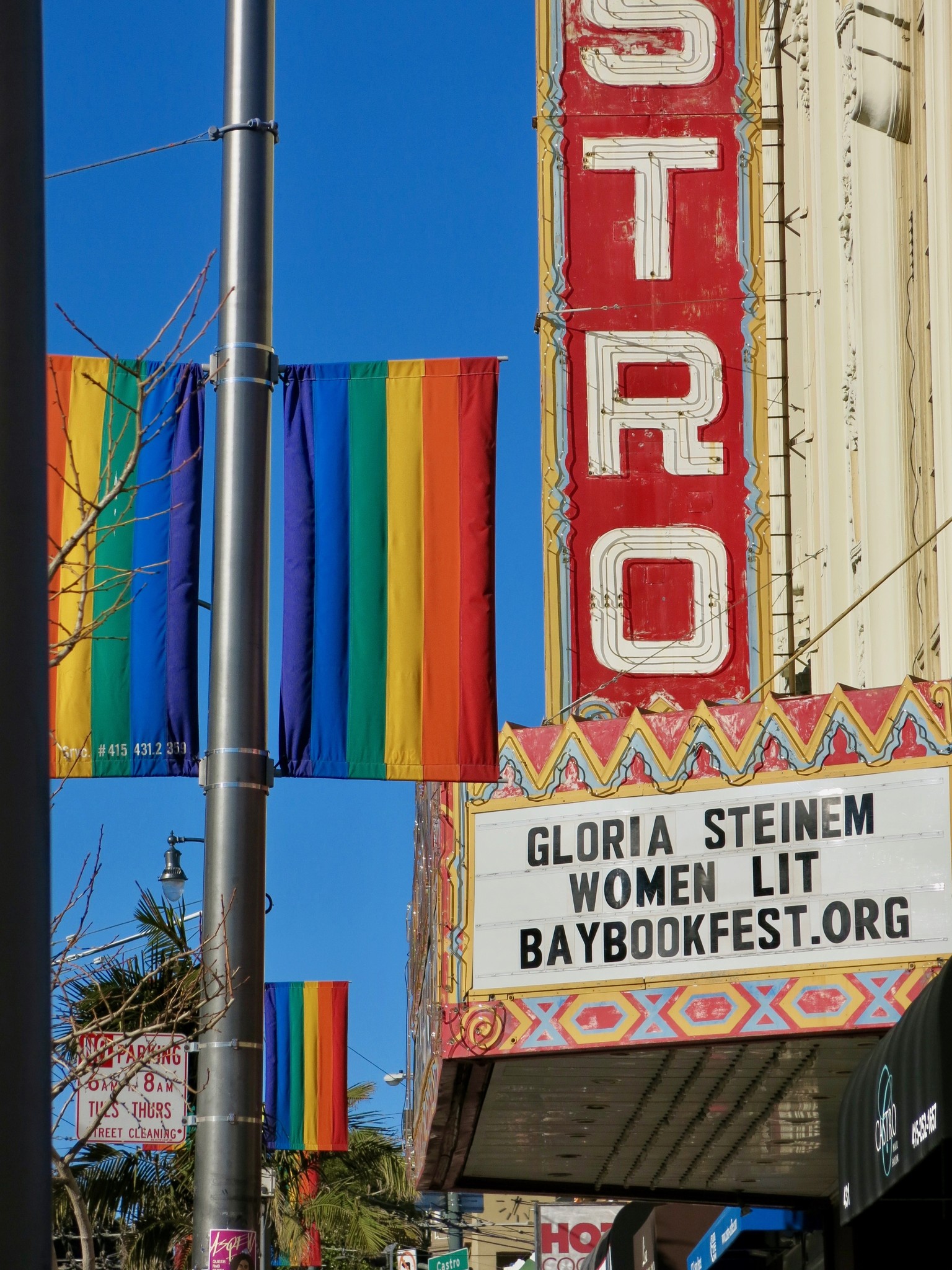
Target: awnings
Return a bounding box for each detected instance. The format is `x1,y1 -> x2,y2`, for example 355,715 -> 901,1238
633,1203 -> 725,1270
833,955 -> 952,1224
579,1201 -> 668,1270
686,1207 -> 806,1270
